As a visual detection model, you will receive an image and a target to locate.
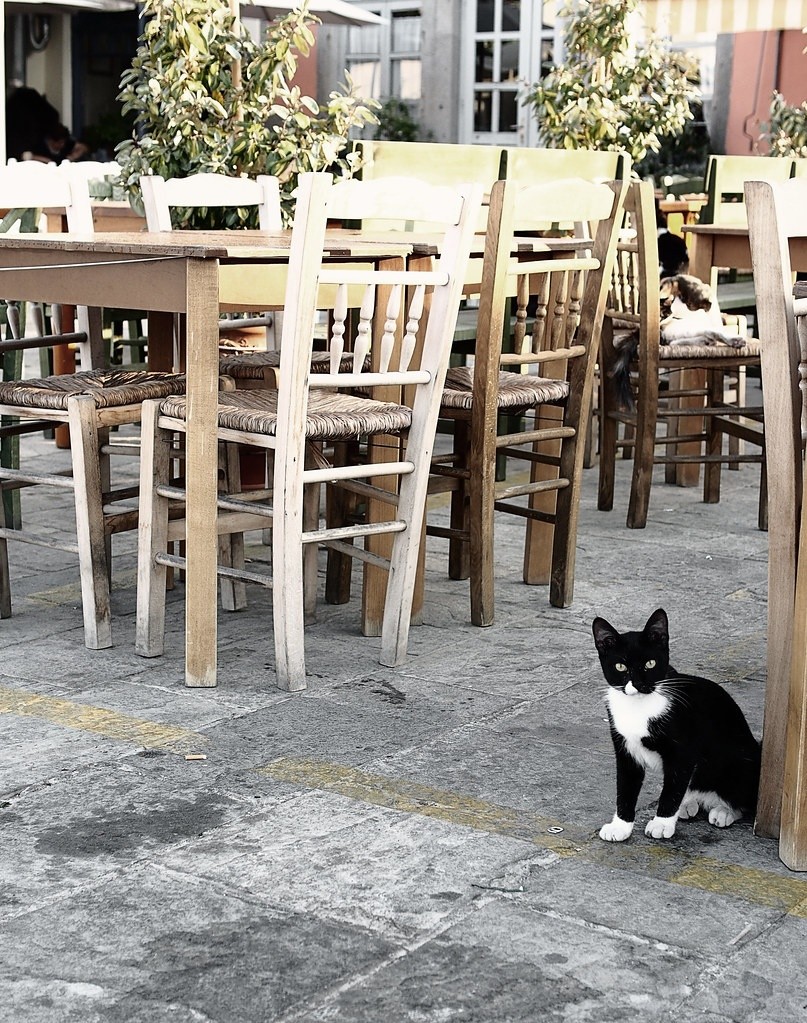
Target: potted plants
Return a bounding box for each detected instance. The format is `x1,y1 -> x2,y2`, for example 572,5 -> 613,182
88,0 -> 384,490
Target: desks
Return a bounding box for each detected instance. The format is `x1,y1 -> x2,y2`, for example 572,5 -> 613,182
0,228 -> 412,689
160,228 -> 595,627
676,221 -> 807,493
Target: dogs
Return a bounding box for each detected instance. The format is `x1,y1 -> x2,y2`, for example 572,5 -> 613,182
606,272 -> 746,415
656,208 -> 690,279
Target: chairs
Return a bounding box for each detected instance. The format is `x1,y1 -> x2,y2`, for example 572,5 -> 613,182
1,139 -> 807,873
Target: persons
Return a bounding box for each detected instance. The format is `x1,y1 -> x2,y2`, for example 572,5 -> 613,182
15,122 -> 88,164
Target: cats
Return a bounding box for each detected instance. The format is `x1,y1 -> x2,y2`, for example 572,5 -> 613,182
591,608 -> 762,843
